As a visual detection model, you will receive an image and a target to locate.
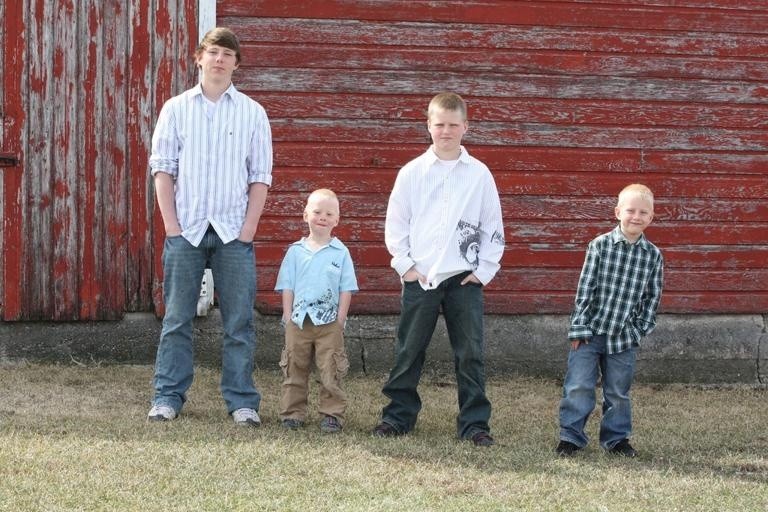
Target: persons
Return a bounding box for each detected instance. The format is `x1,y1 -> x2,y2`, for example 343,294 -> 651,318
367,92 -> 505,446
275,188 -> 359,433
149,27 -> 273,425
556,184 -> 664,457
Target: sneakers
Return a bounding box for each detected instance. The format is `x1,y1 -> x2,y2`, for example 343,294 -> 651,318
284,419 -> 303,429
147,404 -> 176,420
320,416 -> 341,433
610,439 -> 637,457
232,408 -> 261,428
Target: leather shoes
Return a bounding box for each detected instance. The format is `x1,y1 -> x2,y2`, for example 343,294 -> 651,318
472,432 -> 493,445
374,422 -> 399,436
556,440 -> 580,457
